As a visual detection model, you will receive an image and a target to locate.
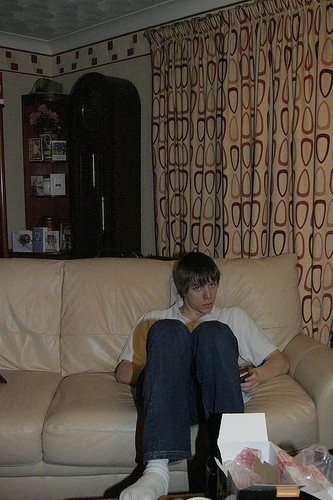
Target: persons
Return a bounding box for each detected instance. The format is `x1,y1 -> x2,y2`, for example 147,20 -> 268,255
115,252 -> 290,500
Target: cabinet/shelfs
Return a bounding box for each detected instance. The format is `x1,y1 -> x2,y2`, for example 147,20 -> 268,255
22,94 -> 79,257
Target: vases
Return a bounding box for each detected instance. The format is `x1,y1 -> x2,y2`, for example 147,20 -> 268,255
39,130 -> 59,158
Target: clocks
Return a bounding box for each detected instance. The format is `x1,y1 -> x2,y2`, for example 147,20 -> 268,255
66,71 -> 142,258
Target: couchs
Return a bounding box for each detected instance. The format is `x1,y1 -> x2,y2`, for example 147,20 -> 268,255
0,252 -> 333,500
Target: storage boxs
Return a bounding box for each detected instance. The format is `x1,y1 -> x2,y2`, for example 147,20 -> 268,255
215,412 -> 333,500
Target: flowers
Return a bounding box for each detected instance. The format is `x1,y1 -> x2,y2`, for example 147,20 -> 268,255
28,103 -> 62,133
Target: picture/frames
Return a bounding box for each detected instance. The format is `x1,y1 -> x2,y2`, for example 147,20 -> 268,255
29,137 -> 44,161
39,133 -> 53,154
